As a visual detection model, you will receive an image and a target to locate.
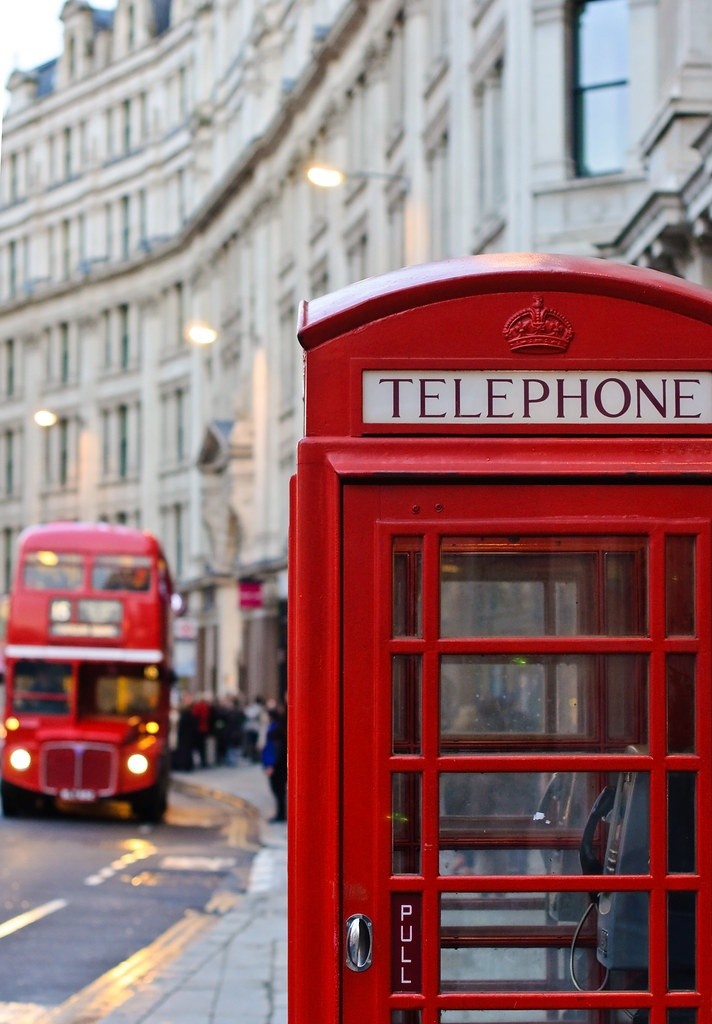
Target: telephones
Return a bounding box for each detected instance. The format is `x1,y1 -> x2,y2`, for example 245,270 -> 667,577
534,772 -> 582,924
578,743 -> 695,971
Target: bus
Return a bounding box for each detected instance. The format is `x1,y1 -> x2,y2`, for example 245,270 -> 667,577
0,525 -> 171,821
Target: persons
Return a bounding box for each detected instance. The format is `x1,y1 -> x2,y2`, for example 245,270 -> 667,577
173,688 -> 287,823
16,665 -> 70,716
104,566 -> 142,593
434,683 -> 548,896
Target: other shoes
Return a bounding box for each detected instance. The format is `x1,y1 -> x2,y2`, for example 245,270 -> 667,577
267,814 -> 284,823
